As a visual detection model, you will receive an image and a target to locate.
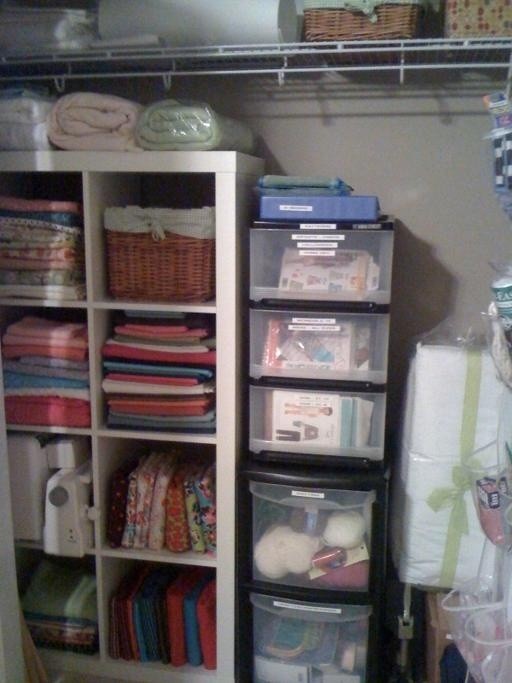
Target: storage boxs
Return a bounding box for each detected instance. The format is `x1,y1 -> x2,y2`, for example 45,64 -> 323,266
248,219 -> 395,305
443,1 -> 511,40
249,307 -> 391,386
245,582 -> 379,683
242,464 -> 383,596
248,380 -> 388,466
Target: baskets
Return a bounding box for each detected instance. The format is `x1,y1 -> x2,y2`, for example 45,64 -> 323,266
104,204 -> 215,303
303,1 -> 429,41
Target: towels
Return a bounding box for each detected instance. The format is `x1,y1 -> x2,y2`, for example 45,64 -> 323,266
1,196 -> 86,300
133,98 -> 255,151
19,560 -> 99,656
253,170 -> 352,199
2,315 -> 91,428
45,92 -> 142,150
1,3 -> 165,56
0,95 -> 53,151
101,310 -> 217,435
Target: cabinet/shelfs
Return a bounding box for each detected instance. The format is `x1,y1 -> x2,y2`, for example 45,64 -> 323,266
0,150 -> 267,681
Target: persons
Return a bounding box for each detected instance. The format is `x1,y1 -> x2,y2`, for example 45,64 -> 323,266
282,402 -> 333,417
